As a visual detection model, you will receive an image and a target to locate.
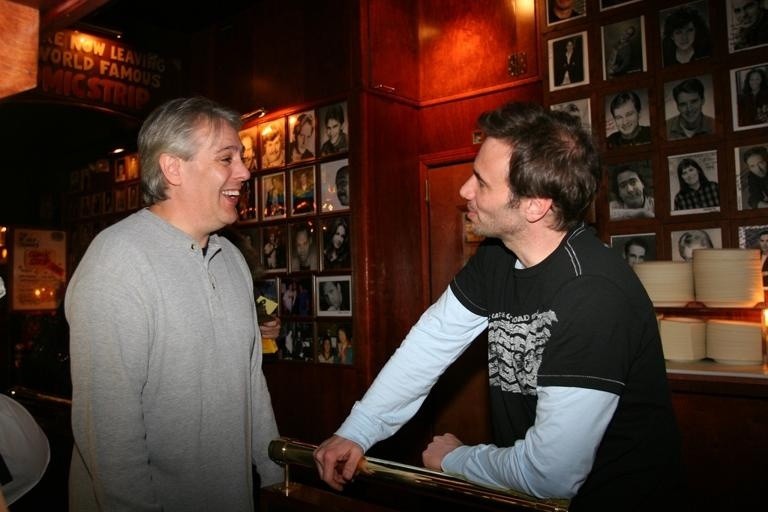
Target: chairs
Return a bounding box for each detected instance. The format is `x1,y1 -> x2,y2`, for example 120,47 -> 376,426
0,394 -> 52,507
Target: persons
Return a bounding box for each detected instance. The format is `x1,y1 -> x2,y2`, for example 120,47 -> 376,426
609,25 -> 636,74
760,231 -> 768,271
667,79 -> 715,138
262,122 -> 285,167
732,0 -> 768,49
624,238 -> 647,262
0,394 -> 52,511
674,158 -> 720,210
610,166 -> 655,219
238,233 -> 259,264
64,98 -> 289,511
265,231 -> 286,269
313,101 -> 678,508
290,113 -> 313,160
664,6 -> 707,65
322,104 -> 348,153
322,281 -> 350,311
318,328 -> 352,363
739,68 -> 768,126
679,230 -> 714,262
283,280 -> 309,314
557,39 -> 579,85
236,184 -> 254,218
336,166 -> 350,204
324,218 -> 350,267
25,232 -> 98,399
283,329 -> 304,356
564,103 -> 579,118
240,133 -> 256,169
84,157 -> 151,235
744,147 -> 768,208
607,90 -> 651,148
266,177 -> 283,216
294,172 -> 313,205
549,0 -> 579,22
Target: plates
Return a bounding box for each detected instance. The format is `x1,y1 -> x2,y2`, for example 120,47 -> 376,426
633,248 -> 765,366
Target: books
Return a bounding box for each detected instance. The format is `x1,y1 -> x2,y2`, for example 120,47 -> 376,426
254,303 -> 278,354
